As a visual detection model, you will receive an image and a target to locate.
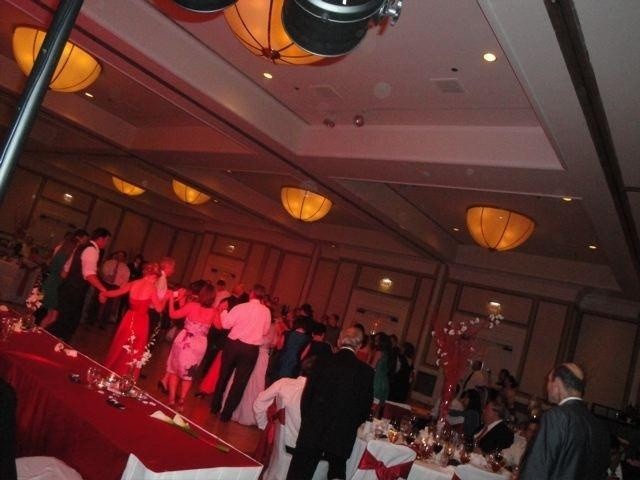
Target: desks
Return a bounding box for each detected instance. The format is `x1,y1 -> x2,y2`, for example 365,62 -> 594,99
0,303 -> 264,480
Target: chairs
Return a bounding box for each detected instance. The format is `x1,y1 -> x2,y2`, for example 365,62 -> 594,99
252,392 -> 330,480
351,439 -> 418,480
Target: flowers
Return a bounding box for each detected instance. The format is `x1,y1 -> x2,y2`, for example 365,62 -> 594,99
430,313 -> 506,430
24,283 -> 44,315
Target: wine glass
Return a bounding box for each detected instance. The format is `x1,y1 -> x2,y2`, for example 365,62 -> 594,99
432,440 -> 444,464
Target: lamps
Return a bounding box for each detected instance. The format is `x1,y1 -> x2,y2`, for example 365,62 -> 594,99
280,186 -> 333,223
112,173 -> 146,197
12,23 -> 102,93
172,178 -> 211,206
466,205 -> 536,252
222,0 -> 325,66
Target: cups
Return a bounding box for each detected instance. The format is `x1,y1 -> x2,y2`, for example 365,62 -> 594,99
118,373 -> 135,394
374,426 -> 400,444
495,452 -> 505,462
86,365 -> 101,384
403,431 -> 417,446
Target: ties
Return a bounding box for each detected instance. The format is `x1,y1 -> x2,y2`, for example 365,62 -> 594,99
473,425 -> 490,443
109,261 -> 120,286
461,371 -> 476,391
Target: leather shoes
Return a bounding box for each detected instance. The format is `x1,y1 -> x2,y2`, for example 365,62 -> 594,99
157,379 -> 208,414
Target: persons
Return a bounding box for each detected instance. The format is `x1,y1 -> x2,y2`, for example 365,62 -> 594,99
513,360 -> 621,480
595,433 -> 640,480
283,324 -> 375,480
32,225 -> 187,391
427,360 -> 519,457
160,281 -> 416,480
0,376 -> 20,480
